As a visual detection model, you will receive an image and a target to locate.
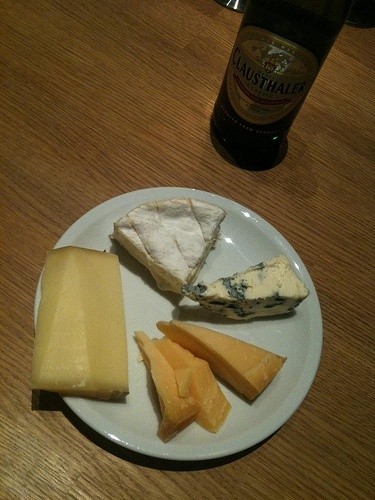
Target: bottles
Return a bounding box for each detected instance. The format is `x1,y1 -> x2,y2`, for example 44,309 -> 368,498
210,0 -> 352,168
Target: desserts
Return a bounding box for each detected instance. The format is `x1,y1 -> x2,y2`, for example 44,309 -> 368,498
182,254 -> 309,321
110,197 -> 226,292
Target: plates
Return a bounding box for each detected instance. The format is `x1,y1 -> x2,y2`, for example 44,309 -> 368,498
34,186 -> 325,462
